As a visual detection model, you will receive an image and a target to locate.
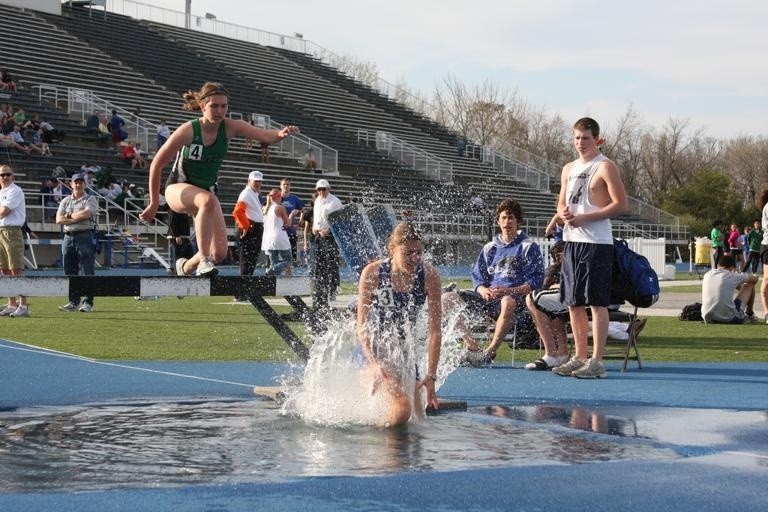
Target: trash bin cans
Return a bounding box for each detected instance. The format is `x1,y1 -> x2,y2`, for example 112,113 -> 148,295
694,242 -> 713,267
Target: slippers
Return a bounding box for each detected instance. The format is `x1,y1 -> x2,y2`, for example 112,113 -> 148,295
525,358 -> 562,371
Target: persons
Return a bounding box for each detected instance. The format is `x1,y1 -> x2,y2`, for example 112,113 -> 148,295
21,220 -> 39,268
351,220 -> 442,428
276,176 -> 304,276
301,192 -> 318,278
301,148 -> 317,171
440,192 -> 625,371
548,116 -> 629,380
243,114 -> 253,150
54,172 -> 100,314
0,68 -> 171,224
698,183 -> 768,326
310,178 -> 342,313
137,80 -> 300,276
260,188 -> 298,280
259,141 -> 271,164
0,163 -> 31,318
457,136 -> 465,156
231,170 -> 263,303
165,209 -> 192,275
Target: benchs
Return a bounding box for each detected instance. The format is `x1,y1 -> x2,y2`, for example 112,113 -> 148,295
1,2 -> 678,274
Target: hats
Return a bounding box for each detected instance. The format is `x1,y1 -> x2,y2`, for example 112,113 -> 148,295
314,179 -> 330,189
71,173 -> 85,182
248,171 -> 264,181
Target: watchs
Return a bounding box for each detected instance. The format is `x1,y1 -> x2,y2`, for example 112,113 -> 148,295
425,373 -> 437,382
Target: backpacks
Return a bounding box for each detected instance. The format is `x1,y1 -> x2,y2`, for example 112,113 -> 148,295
612,236 -> 660,308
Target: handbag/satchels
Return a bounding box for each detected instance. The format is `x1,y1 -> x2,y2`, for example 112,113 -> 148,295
681,302 -> 705,321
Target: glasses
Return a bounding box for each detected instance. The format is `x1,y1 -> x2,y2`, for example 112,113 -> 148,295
317,188 -> 328,191
0,173 -> 13,177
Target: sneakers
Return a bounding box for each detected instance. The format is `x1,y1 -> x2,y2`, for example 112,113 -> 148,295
765,313 -> 768,325
196,257 -> 219,276
453,347 -> 496,367
79,301 -> 96,313
571,358 -> 608,380
58,302 -> 80,312
233,296 -> 251,303
175,258 -> 192,300
0,306 -> 18,316
10,306 -> 32,317
552,356 -> 588,377
743,311 -> 758,321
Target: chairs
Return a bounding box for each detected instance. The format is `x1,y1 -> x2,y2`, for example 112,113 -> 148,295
454,294 -> 540,369
554,272 -> 644,374
